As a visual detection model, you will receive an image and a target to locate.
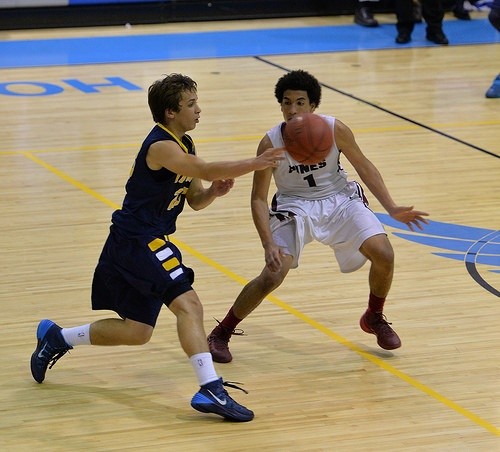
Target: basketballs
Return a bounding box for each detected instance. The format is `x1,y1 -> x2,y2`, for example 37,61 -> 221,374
284,113 -> 333,165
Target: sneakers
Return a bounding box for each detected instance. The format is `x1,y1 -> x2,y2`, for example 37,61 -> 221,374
207,317 -> 244,363
360,310 -> 401,350
486,74 -> 500,98
191,377 -> 254,422
31,319 -> 73,384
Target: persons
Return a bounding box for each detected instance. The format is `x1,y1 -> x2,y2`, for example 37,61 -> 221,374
206,69 -> 430,363
353,0 -> 500,98
30,73 -> 287,423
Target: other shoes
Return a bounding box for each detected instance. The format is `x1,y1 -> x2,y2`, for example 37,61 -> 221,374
430,32 -> 449,44
354,7 -> 378,27
396,31 -> 411,44
